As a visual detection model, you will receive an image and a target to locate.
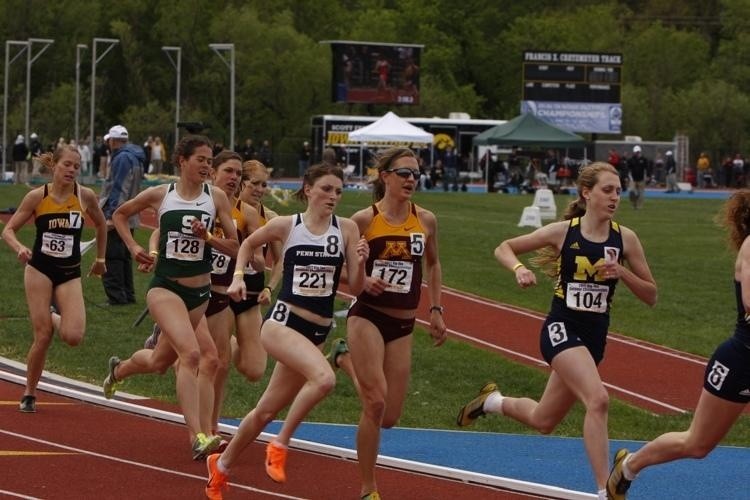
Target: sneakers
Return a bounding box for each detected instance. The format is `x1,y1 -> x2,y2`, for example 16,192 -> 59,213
325,336 -> 350,375
18,394 -> 37,413
97,299 -> 137,308
264,441 -> 290,485
605,447 -> 633,500
49,305 -> 57,337
190,431 -> 229,462
204,453 -> 230,500
144,320 -> 162,351
102,355 -> 125,401
358,490 -> 382,500
455,382 -> 500,427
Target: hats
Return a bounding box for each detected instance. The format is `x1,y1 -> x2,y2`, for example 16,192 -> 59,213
632,145 -> 641,152
103,125 -> 129,142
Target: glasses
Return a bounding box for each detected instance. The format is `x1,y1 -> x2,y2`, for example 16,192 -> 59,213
386,166 -> 421,181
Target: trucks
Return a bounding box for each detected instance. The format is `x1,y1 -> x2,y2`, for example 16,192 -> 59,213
310,111 -> 511,176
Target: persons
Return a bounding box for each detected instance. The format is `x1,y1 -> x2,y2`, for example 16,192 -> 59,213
340,46 -> 420,104
2,126 -> 750,499
